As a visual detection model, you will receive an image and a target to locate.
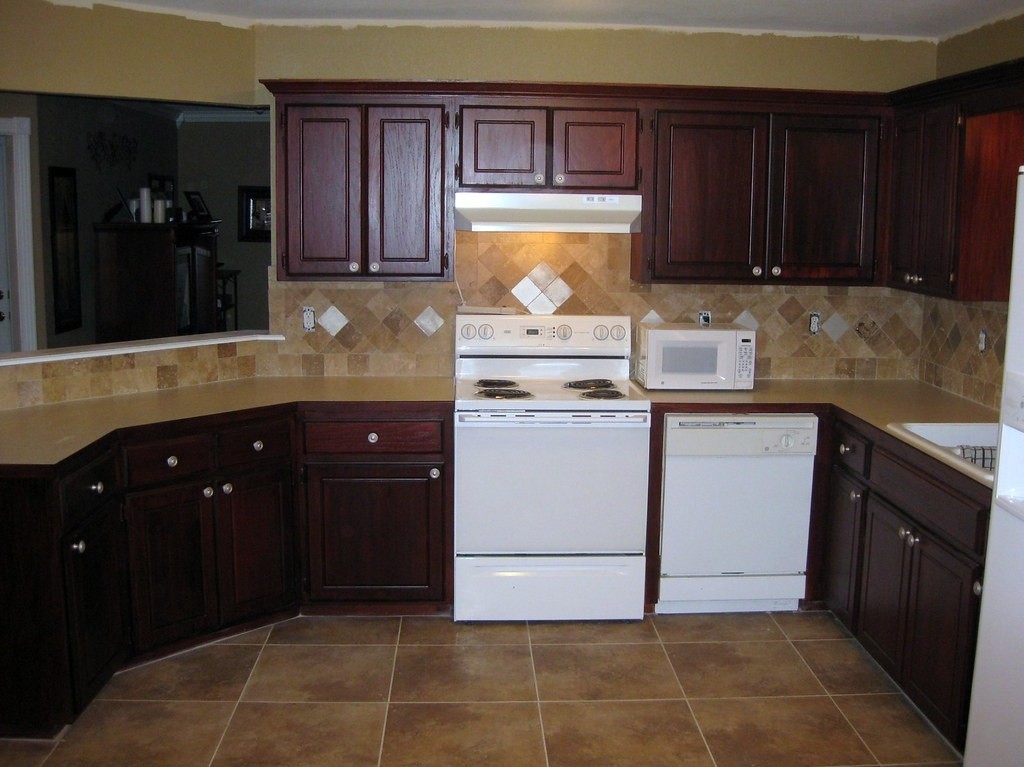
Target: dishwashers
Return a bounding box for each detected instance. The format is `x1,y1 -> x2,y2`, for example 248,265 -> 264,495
656,410 -> 819,617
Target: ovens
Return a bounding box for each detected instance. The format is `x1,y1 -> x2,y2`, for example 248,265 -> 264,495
452,411 -> 651,621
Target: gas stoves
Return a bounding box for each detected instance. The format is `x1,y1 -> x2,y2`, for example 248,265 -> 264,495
454,314 -> 652,411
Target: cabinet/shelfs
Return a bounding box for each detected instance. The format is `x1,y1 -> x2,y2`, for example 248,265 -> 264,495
859,427 -> 1021,760
631,84 -> 888,289
259,78 -> 453,283
454,79 -> 641,232
0,429 -> 128,741
887,57 -> 1024,303
116,402 -> 299,659
92,221 -> 220,344
826,403 -> 878,637
299,402 -> 454,619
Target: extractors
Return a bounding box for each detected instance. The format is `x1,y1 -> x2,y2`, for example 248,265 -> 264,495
455,191 -> 643,234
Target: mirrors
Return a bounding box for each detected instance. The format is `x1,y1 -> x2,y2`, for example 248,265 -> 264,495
47,167 -> 86,335
0,89 -> 270,357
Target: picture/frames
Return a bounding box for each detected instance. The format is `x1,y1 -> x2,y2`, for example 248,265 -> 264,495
238,184 -> 271,243
182,190 -> 213,222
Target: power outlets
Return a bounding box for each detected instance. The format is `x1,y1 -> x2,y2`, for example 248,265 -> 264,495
809,312 -> 820,335
303,307 -> 315,332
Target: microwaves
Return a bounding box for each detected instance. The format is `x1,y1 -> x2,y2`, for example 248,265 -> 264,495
634,322 -> 758,392
